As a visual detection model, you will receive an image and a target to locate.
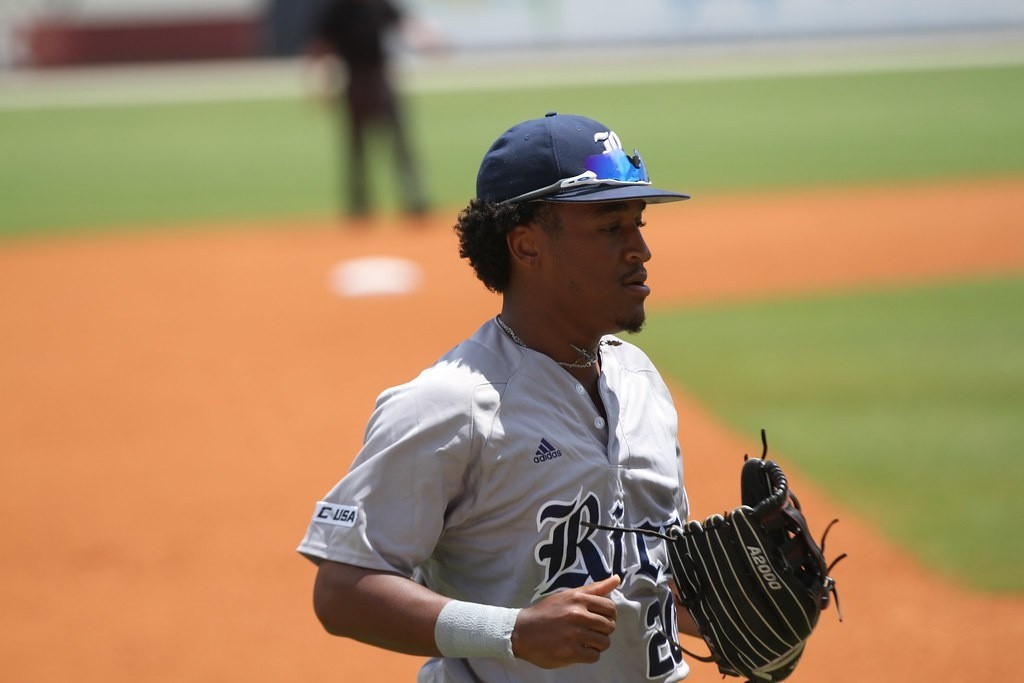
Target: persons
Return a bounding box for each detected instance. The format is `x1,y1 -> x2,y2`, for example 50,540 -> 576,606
282,0 -> 441,215
296,114 -> 833,683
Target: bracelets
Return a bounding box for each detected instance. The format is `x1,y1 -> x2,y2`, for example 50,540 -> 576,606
434,600 -> 521,664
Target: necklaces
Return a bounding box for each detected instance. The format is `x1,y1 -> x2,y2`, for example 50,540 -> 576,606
495,315 -> 602,368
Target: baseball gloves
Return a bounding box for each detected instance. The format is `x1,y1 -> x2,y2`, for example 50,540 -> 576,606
583,427 -> 847,679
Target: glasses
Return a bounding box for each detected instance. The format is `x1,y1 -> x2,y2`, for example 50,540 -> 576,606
499,148 -> 653,206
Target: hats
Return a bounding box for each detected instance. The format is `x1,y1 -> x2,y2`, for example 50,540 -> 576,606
475,112 -> 690,204
